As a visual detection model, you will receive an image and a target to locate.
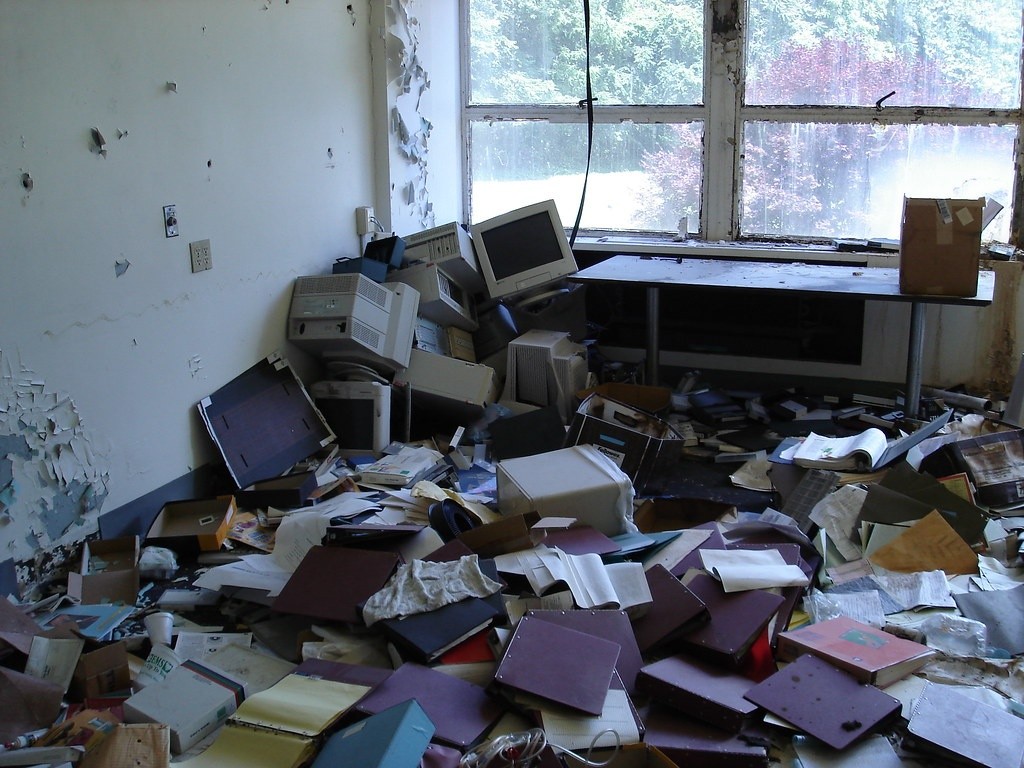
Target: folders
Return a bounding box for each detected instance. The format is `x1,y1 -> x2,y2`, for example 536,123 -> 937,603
268,511 -> 940,768
767,408 -> 957,474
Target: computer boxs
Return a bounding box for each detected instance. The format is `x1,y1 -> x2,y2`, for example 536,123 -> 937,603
380,220 -> 519,426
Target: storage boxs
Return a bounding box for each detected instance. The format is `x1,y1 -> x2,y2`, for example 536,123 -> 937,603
305,476 -> 360,505
38,620 -> 131,698
122,658 -> 250,754
414,317 -> 449,356
148,494 -> 236,553
898,192 -> 1004,296
450,327 -> 477,363
563,391 -> 688,496
496,443 -> 636,536
330,236 -> 407,281
921,427 -> 1024,508
67,534 -> 142,606
233,470 -> 317,506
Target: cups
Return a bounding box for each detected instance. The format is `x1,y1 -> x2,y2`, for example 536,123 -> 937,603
133,645 -> 180,687
144,612 -> 174,647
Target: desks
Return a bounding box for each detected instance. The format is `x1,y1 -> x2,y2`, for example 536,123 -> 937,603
567,254 -> 996,424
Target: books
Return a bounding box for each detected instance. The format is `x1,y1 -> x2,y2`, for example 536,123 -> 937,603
744,653 -> 903,754
379,597 -> 499,665
169,672 -> 373,768
1,373 -> 1022,767
774,614 -> 935,689
697,546 -> 809,594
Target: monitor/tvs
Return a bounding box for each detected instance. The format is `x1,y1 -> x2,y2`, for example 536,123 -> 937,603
310,381 -> 391,460
288,273 -> 420,384
470,199 -> 578,307
499,329 -> 589,416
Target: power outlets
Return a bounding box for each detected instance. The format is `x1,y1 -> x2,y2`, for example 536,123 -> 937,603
190,238 -> 214,273
